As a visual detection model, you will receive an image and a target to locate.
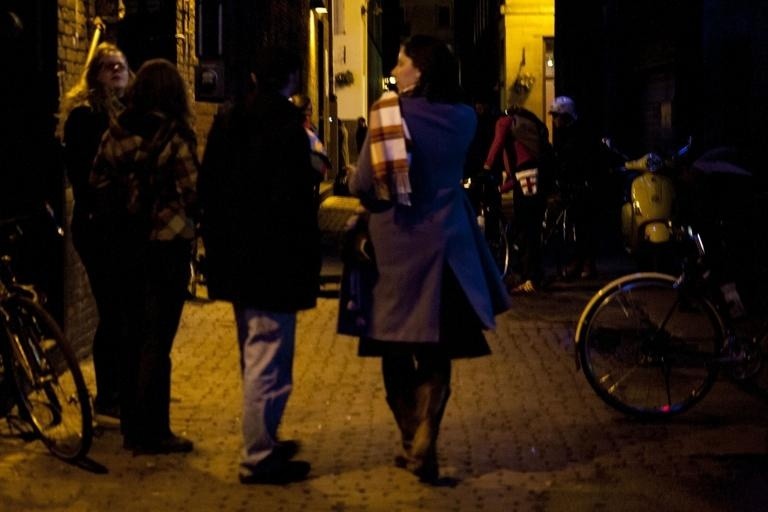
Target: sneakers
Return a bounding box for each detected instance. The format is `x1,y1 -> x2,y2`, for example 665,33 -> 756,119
509,280 -> 539,297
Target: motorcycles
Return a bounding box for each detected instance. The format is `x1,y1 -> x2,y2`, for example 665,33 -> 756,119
614,133 -> 696,269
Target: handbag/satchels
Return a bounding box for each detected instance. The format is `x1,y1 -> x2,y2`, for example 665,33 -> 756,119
86,160 -> 162,265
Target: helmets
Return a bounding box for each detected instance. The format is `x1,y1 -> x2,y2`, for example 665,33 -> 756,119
547,95 -> 577,116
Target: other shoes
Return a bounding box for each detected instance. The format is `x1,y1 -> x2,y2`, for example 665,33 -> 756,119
124,431 -> 194,455
263,440 -> 301,461
393,453 -> 439,483
238,460 -> 313,485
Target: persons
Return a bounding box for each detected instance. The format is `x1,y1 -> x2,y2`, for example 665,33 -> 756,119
87,59 -> 198,453
63,43 -> 139,419
468,94 -> 767,319
347,32 -> 510,476
197,42 -> 321,484
333,117 -> 369,197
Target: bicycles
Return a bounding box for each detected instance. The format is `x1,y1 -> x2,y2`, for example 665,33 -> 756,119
0,251 -> 106,464
460,149 -> 597,286
566,206 -> 766,424
